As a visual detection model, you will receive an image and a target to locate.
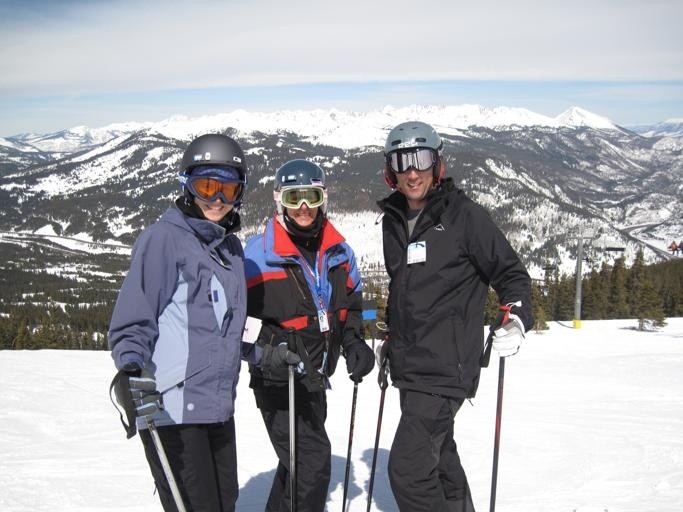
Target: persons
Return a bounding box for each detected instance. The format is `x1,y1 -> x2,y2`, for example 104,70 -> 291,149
373,121 -> 536,511
668,241 -> 679,256
242,159 -> 375,511
677,240 -> 683,254
106,135 -> 306,512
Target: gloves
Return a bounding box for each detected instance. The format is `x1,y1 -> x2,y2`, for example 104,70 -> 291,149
491,314 -> 526,357
257,327 -> 314,392
343,338 -> 376,383
110,363 -> 162,440
375,339 -> 390,375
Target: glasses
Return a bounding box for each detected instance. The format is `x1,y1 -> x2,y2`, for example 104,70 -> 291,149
386,147 -> 436,173
279,185 -> 325,209
186,174 -> 243,205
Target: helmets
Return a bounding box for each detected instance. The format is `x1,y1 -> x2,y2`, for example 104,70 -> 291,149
180,134 -> 247,173
384,121 -> 443,158
274,159 -> 326,195
